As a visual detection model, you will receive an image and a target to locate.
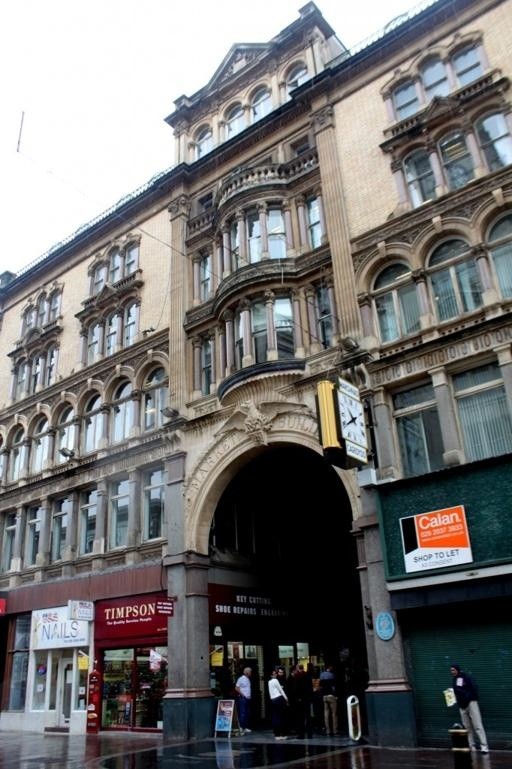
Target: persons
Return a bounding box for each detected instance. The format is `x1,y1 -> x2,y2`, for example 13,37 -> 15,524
339,647 -> 355,687
266,662 -> 339,742
447,662 -> 491,755
232,666 -> 258,735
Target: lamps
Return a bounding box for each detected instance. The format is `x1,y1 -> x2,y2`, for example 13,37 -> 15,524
335,335 -> 361,354
159,406 -> 181,420
57,447 -> 76,459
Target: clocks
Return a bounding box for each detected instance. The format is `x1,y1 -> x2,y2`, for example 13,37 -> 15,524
332,385 -> 370,451
